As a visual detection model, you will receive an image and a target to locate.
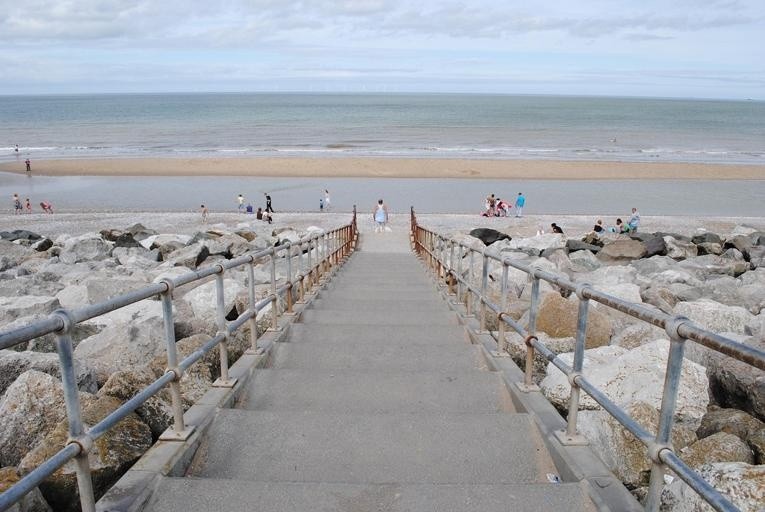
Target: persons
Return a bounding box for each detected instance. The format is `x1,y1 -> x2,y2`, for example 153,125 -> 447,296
262,209 -> 272,224
237,194 -> 245,216
13,194 -> 53,215
24,158 -> 31,171
514,192 -> 525,217
257,207 -> 263,220
480,193 -> 509,217
201,205 -> 209,223
264,193 -> 274,213
15,144 -> 18,152
246,203 -> 253,214
551,223 -> 563,233
373,199 -> 388,233
325,190 -> 330,213
320,199 -> 324,211
593,208 -> 640,234
